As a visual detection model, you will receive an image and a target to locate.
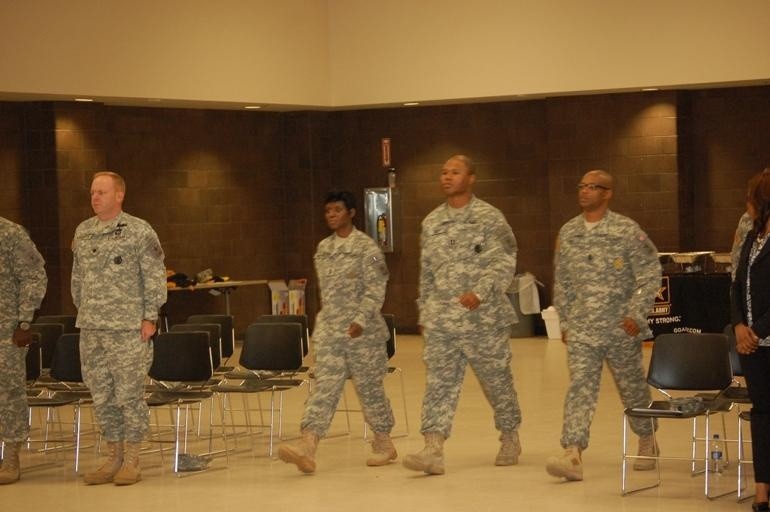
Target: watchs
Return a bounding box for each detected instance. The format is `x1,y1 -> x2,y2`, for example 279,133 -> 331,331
16,320 -> 33,332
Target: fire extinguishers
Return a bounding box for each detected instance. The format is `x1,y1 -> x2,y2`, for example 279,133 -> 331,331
377,213 -> 389,249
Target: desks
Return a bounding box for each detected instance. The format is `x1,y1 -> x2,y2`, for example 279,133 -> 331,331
161,278 -> 268,317
641,270 -> 734,344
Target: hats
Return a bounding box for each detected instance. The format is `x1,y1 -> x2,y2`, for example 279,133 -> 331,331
668,395 -> 705,413
196,267 -> 213,284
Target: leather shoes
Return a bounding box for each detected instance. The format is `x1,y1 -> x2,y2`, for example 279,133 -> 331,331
751,488 -> 770,512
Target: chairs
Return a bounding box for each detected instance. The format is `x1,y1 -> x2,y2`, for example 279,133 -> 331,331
321,314 -> 400,439
146,314 -> 313,473
19,322 -> 102,474
733,347 -> 755,501
687,323 -> 752,476
618,332 -> 748,499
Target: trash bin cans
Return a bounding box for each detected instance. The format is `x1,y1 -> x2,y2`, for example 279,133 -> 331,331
505,272 -> 540,338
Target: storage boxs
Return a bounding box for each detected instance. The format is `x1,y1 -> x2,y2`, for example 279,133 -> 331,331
266,277 -> 308,314
541,305 -> 564,342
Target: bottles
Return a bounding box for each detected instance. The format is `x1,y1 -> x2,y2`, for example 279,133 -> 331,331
710,434 -> 723,476
388,167 -> 396,187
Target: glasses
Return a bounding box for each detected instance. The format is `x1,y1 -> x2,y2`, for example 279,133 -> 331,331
576,182 -> 611,191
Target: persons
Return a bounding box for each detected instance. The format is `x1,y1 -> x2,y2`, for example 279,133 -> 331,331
273,186 -> 398,475
725,207 -> 760,418
543,168 -> 663,481
727,166 -> 770,512
0,214 -> 47,484
402,152 -> 525,476
66,169 -> 171,486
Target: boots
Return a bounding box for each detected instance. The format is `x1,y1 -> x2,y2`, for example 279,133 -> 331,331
111,438 -> 143,486
546,444 -> 584,482
402,429 -> 446,476
366,431 -> 398,466
494,428 -> 522,467
633,431 -> 660,471
0,440 -> 22,484
278,430 -> 320,474
83,440 -> 124,484
